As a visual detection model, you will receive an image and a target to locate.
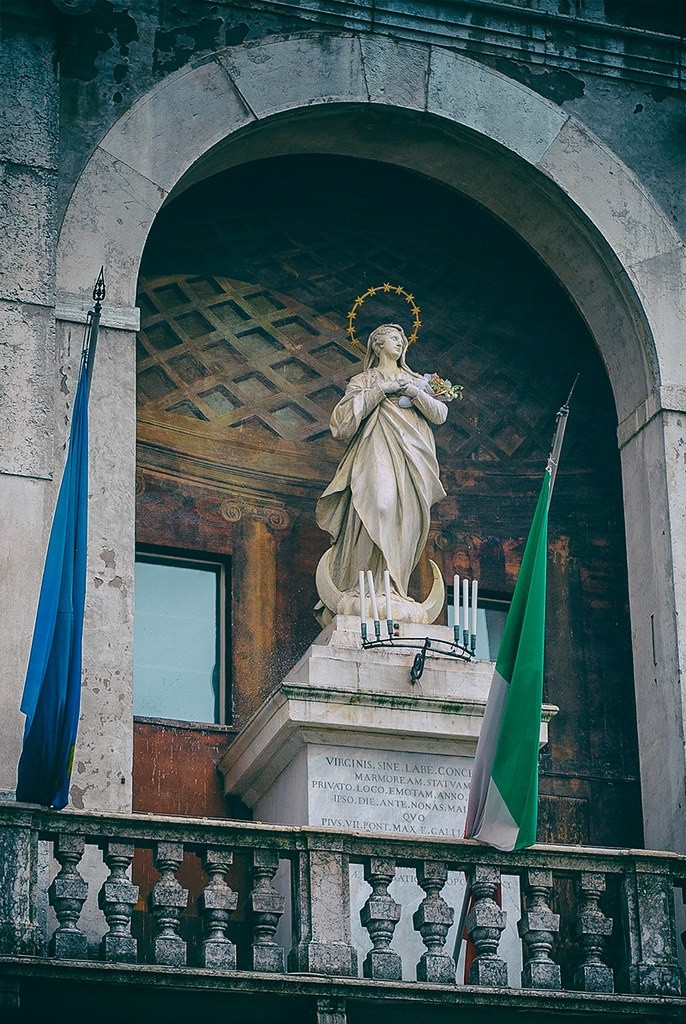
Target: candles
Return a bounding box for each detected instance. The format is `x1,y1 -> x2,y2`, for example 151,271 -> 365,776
382,570 -> 393,620
367,570 -> 380,621
471,578 -> 478,636
463,578 -> 469,630
358,570 -> 367,624
453,573 -> 460,626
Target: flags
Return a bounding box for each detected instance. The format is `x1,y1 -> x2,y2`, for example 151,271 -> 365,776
15,367 -> 89,810
463,457 -> 549,852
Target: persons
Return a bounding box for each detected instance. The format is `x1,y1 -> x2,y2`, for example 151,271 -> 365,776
313,324 -> 448,621
